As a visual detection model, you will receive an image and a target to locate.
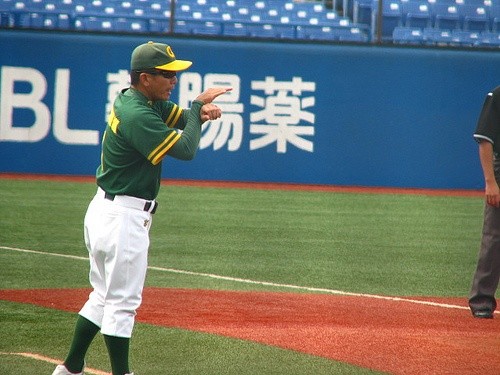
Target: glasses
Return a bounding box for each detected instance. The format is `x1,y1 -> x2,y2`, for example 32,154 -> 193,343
147,70 -> 177,78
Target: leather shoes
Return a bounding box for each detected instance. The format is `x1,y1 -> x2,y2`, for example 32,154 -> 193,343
473,310 -> 494,319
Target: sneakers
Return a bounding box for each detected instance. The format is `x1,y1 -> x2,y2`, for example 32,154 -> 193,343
52,361 -> 86,375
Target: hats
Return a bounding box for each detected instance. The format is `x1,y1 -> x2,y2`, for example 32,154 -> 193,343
131,41 -> 193,71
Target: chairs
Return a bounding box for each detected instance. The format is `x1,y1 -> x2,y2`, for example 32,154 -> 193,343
354,0 -> 500,49
0,0 -> 370,42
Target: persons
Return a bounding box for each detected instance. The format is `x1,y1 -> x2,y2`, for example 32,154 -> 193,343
48,40 -> 234,375
468,82 -> 500,319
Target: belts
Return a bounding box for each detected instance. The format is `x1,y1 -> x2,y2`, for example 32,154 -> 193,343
98,187 -> 158,214
